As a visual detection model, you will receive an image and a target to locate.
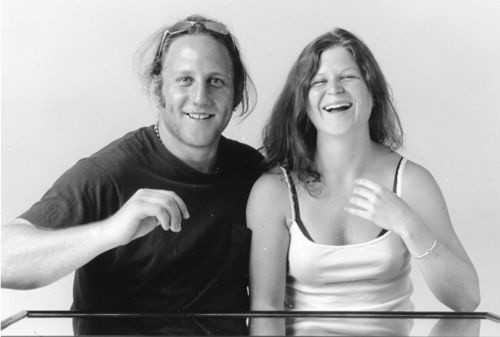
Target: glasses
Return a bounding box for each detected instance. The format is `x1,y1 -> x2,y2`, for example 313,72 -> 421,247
154,19 -> 237,61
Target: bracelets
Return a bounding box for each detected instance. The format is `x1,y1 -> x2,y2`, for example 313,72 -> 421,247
413,239 -> 438,260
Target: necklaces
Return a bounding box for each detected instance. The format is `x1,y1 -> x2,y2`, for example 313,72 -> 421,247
154,122 -> 220,174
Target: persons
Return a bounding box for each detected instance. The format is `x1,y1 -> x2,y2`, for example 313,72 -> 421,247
1,14 -> 267,337
245,27 -> 482,337
